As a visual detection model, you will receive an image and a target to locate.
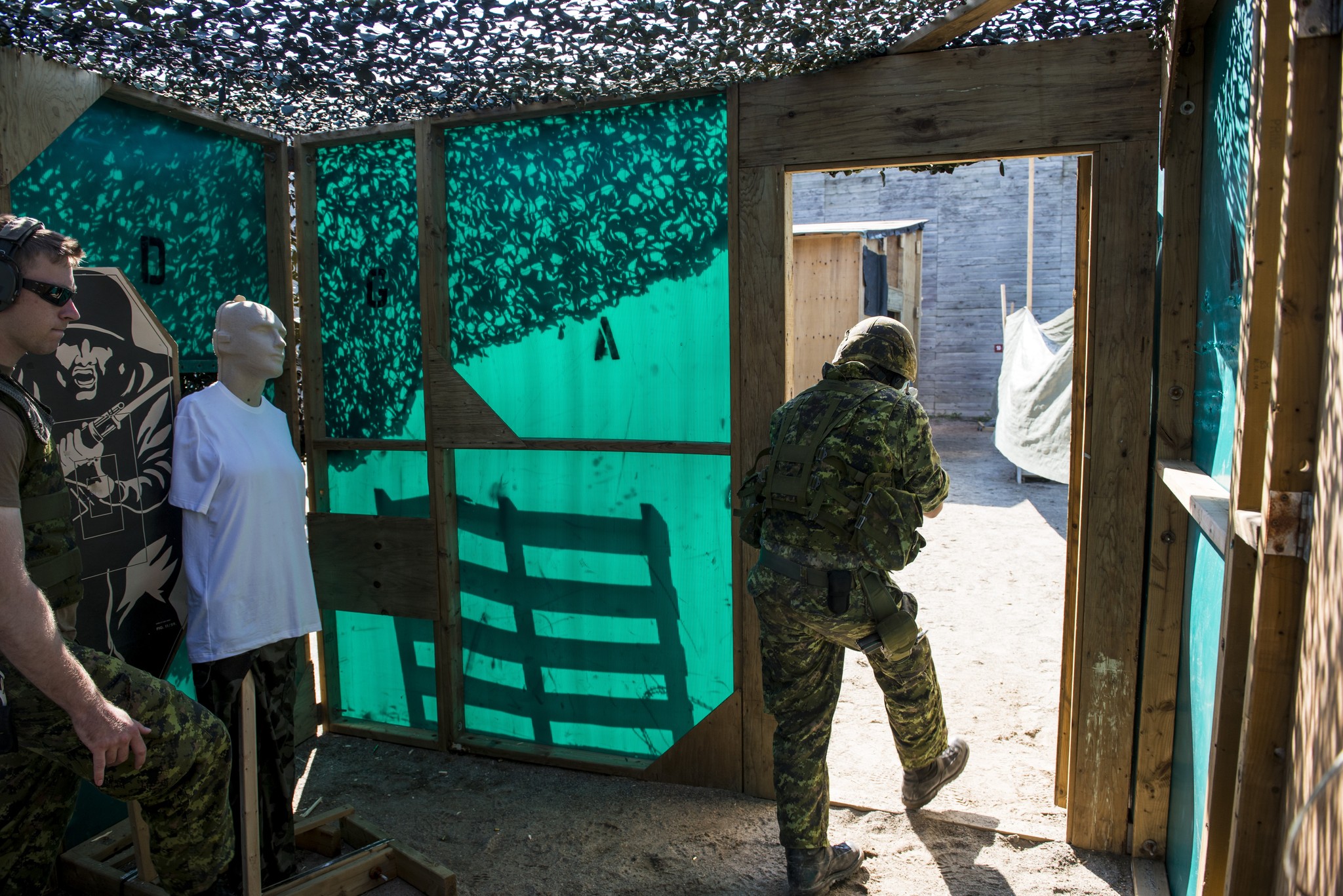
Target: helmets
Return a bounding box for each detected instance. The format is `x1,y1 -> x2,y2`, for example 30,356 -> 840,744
830,316 -> 918,383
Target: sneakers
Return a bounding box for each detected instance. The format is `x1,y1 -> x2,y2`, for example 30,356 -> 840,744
901,737 -> 969,808
785,840 -> 865,896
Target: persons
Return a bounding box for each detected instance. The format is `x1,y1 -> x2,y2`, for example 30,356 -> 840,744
0,209 -> 237,896
736,315 -> 972,896
170,294 -> 328,887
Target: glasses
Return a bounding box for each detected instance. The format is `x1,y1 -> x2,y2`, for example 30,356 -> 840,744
22,277 -> 79,307
